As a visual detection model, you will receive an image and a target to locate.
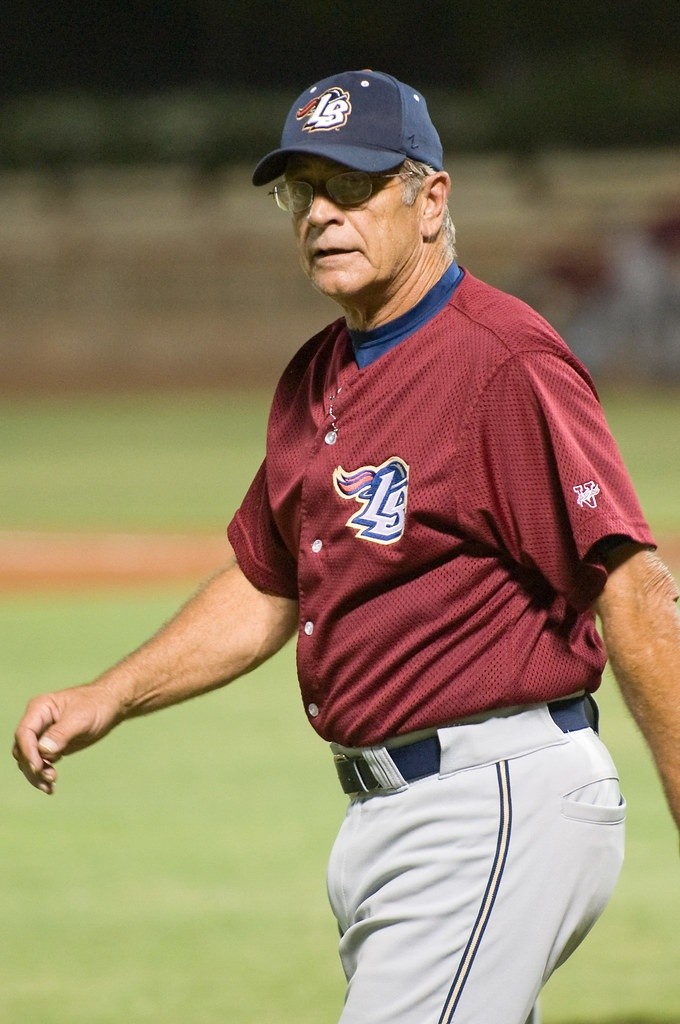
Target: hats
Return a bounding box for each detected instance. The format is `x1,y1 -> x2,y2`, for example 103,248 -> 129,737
252,69 -> 443,186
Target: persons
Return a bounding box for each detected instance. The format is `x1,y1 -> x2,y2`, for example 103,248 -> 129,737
13,70 -> 680,1023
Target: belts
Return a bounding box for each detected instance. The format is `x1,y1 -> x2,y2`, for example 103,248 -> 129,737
333,697 -> 590,792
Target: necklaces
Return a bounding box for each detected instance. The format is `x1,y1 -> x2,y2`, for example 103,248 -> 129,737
324,388 -> 342,446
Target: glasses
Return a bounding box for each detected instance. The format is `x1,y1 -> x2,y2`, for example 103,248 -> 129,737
268,171 -> 418,211
